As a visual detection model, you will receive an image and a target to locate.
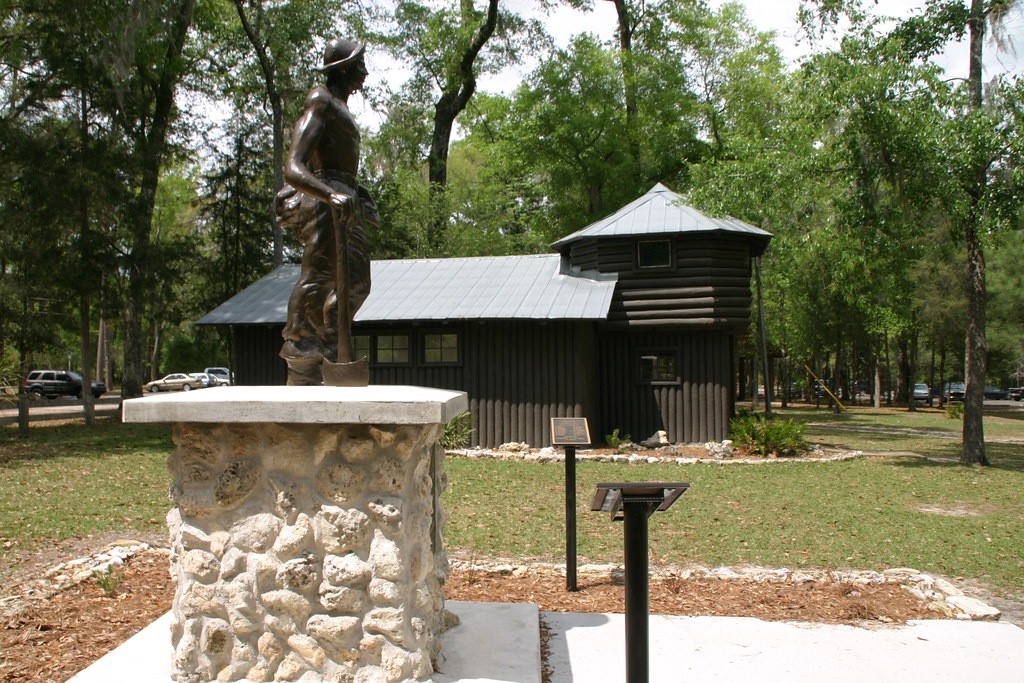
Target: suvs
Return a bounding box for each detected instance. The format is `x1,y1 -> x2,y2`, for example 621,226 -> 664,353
205,367 -> 236,380
25,370 -> 107,400
944,382 -> 967,403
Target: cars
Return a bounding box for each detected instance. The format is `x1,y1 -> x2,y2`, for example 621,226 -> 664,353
188,373 -> 216,389
914,384 -> 931,403
145,373 -> 202,391
814,379 -> 832,397
208,374 -> 233,387
982,386 -> 1008,400
757,382 -> 802,399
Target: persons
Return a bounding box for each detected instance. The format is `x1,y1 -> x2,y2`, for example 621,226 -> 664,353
273,38 -> 378,389
